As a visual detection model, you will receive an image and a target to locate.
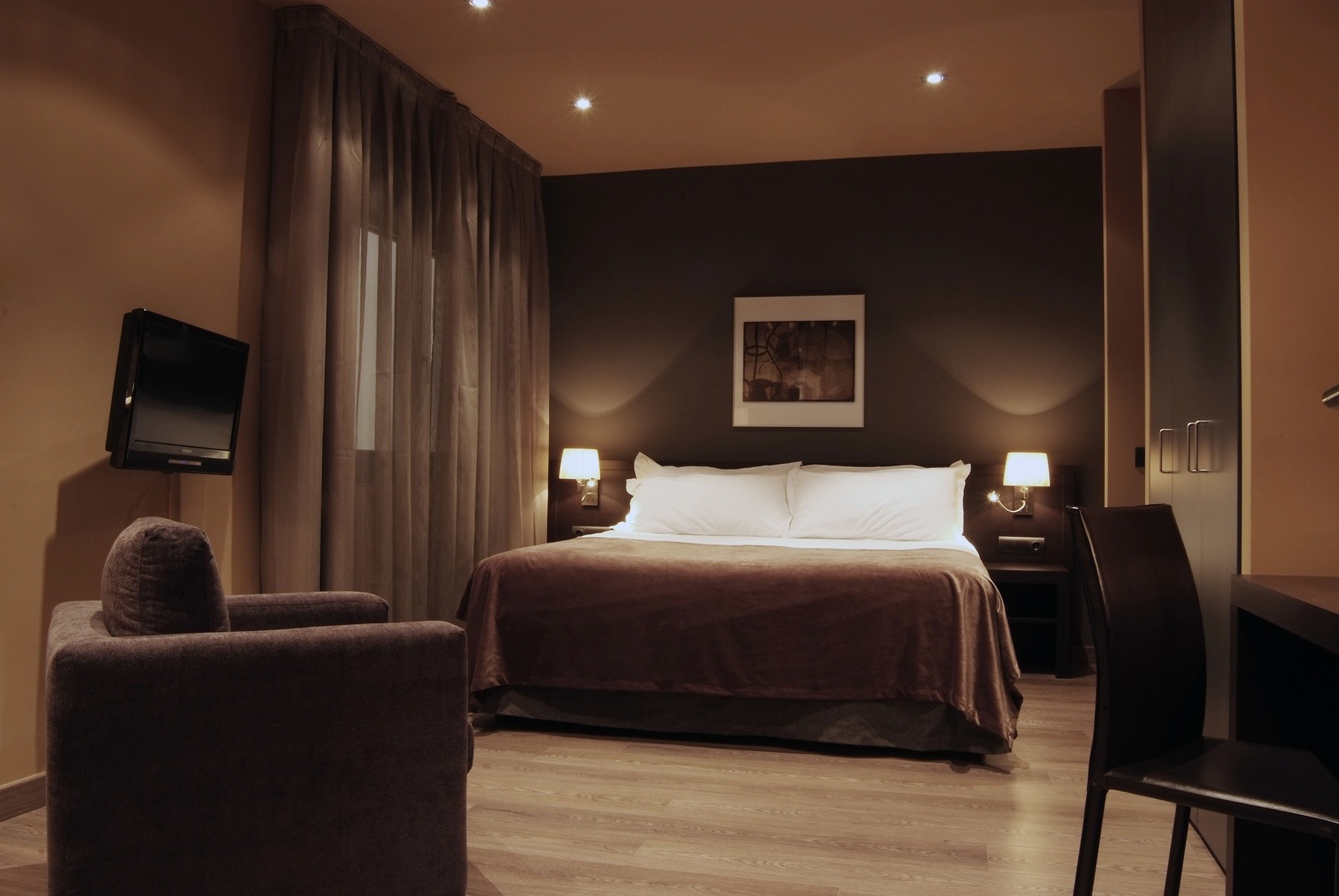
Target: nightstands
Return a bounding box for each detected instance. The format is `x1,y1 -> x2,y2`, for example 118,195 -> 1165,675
983,559 -> 1075,679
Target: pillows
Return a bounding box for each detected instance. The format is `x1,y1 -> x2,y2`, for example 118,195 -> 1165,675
611,452 -> 803,539
784,460 -> 972,542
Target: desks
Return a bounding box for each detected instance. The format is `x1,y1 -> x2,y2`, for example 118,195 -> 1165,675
1225,572 -> 1339,896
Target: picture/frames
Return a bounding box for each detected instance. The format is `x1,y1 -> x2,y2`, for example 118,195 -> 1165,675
725,290 -> 870,434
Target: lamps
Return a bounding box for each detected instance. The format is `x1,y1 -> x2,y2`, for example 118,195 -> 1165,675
985,449 -> 1051,517
557,446 -> 602,495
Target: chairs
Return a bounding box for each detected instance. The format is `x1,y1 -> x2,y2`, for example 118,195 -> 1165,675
1064,497 -> 1339,896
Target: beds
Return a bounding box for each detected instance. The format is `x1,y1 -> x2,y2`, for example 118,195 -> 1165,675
469,456 -> 995,768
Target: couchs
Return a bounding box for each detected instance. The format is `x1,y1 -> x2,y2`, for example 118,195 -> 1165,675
42,589 -> 478,896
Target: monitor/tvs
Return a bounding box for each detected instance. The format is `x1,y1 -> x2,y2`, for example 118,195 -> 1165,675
105,307 -> 250,476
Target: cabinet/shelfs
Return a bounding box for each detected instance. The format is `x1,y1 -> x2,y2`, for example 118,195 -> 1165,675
1136,0 -> 1245,875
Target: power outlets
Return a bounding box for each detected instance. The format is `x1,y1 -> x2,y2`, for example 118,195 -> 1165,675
579,488 -> 599,507
1011,498 -> 1036,516
